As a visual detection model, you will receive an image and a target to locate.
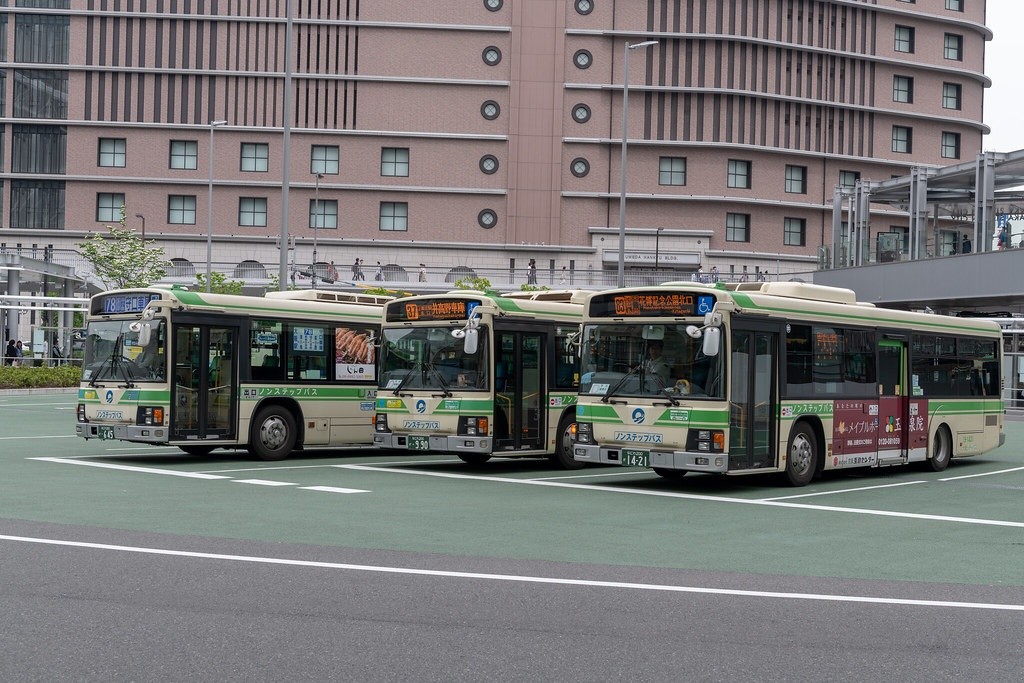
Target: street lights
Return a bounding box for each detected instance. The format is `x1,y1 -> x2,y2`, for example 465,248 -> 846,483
313,171 -> 325,290
655,227 -> 664,271
617,40 -> 658,288
135,212 -> 145,257
206,120 -> 227,293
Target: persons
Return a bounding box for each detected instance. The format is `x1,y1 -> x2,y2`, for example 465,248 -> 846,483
691,266 -> 703,282
997,227 -> 1006,250
758,270 -> 768,282
559,267 -> 566,284
6,339 -> 23,366
419,264 -> 427,282
528,261 -> 538,284
639,340 -> 670,390
711,266 -> 718,283
352,258 -> 365,281
136,338 -> 158,369
376,261 -> 382,281
52,340 -> 64,367
963,234 -> 971,253
741,271 -> 749,282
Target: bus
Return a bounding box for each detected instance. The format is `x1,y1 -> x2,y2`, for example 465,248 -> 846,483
372,290 -> 647,469
573,280 -> 1007,487
74,283 -> 414,458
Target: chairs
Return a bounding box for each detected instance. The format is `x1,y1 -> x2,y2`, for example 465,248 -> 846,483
262,356 -> 280,367
